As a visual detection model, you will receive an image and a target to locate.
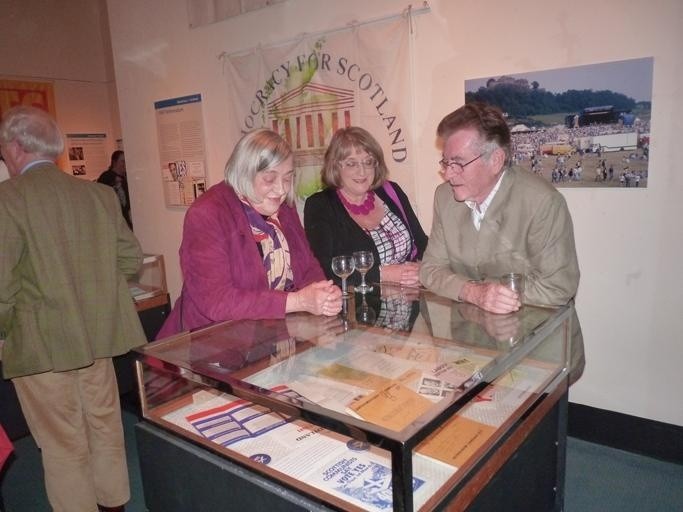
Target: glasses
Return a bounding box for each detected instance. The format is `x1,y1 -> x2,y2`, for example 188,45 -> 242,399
438,152 -> 485,176
338,158 -> 378,169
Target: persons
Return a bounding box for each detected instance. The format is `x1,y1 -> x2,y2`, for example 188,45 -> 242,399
153,128 -> 345,341
417,102 -> 586,391
305,124 -> 430,287
337,283 -> 426,372
69,146 -> 88,176
427,293 -> 521,349
187,316 -> 347,405
2,107 -> 149,512
167,160 -> 180,181
506,114 -> 649,187
94,149 -> 135,232
418,378 -> 456,398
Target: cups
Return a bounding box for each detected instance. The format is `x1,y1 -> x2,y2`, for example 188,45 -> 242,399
499,273 -> 524,308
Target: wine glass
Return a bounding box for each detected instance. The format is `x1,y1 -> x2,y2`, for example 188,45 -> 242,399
331,254 -> 354,299
339,292 -> 376,329
352,250 -> 375,293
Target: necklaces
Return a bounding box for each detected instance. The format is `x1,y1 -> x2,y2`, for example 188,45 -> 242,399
335,186 -> 377,216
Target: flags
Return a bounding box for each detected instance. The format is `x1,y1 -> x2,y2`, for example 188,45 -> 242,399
222,11 -> 419,227
